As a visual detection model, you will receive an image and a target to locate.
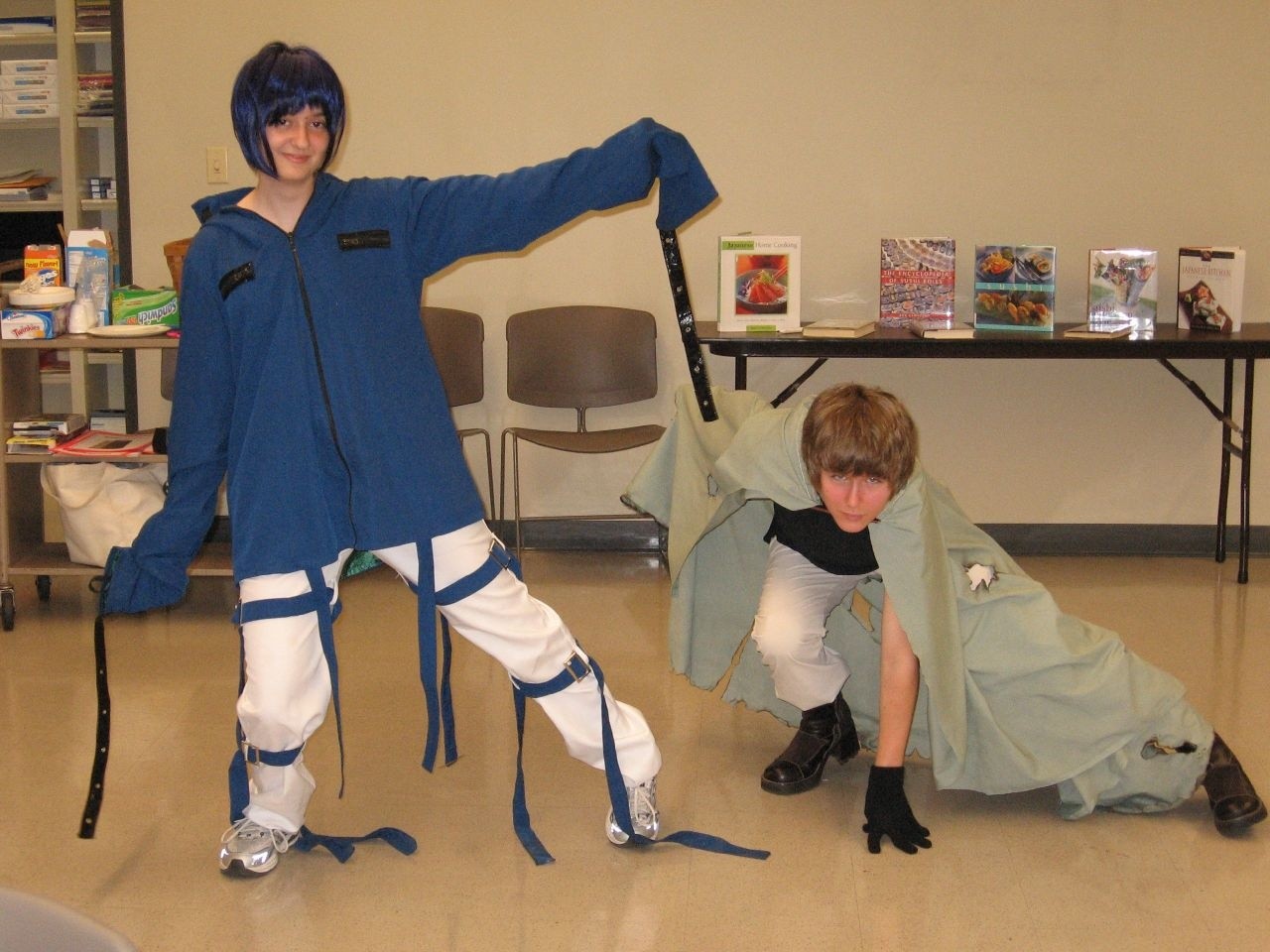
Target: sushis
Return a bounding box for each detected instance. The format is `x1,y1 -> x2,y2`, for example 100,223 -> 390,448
1024,253 -> 1051,275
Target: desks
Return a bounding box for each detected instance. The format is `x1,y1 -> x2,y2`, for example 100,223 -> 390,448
695,321 -> 1270,585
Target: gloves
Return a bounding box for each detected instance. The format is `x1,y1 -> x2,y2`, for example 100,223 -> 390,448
861,764 -> 932,854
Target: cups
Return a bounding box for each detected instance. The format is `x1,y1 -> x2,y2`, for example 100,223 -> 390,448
69,299 -> 95,333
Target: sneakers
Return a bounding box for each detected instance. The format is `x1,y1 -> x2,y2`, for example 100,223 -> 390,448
604,780 -> 658,847
220,818 -> 300,878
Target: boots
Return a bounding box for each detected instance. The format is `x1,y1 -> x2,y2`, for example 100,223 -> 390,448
760,691 -> 862,795
1200,729 -> 1267,833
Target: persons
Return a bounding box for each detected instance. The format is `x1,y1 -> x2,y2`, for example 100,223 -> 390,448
105,41 -> 720,876
674,384 -> 1267,854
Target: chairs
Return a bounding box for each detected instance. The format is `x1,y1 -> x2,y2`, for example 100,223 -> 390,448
500,305 -> 669,573
419,306 -> 496,536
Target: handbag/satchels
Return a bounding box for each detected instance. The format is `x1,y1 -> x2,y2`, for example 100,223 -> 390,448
38,462 -> 171,568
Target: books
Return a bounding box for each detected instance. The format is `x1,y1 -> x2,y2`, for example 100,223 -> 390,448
716,234 -> 1247,340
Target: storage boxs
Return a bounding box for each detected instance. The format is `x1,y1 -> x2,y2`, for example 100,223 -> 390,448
0,223 -> 180,340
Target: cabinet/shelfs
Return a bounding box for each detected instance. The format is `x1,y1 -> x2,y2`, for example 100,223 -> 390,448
0,294 -> 234,631
0,0 -> 138,435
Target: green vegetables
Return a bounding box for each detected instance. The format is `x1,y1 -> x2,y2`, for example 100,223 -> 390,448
757,270 -> 774,284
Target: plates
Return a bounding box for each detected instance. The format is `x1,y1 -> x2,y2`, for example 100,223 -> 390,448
87,324 -> 172,337
8,286 -> 75,310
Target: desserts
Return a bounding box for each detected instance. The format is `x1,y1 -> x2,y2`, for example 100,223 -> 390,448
1193,292 -> 1226,331
